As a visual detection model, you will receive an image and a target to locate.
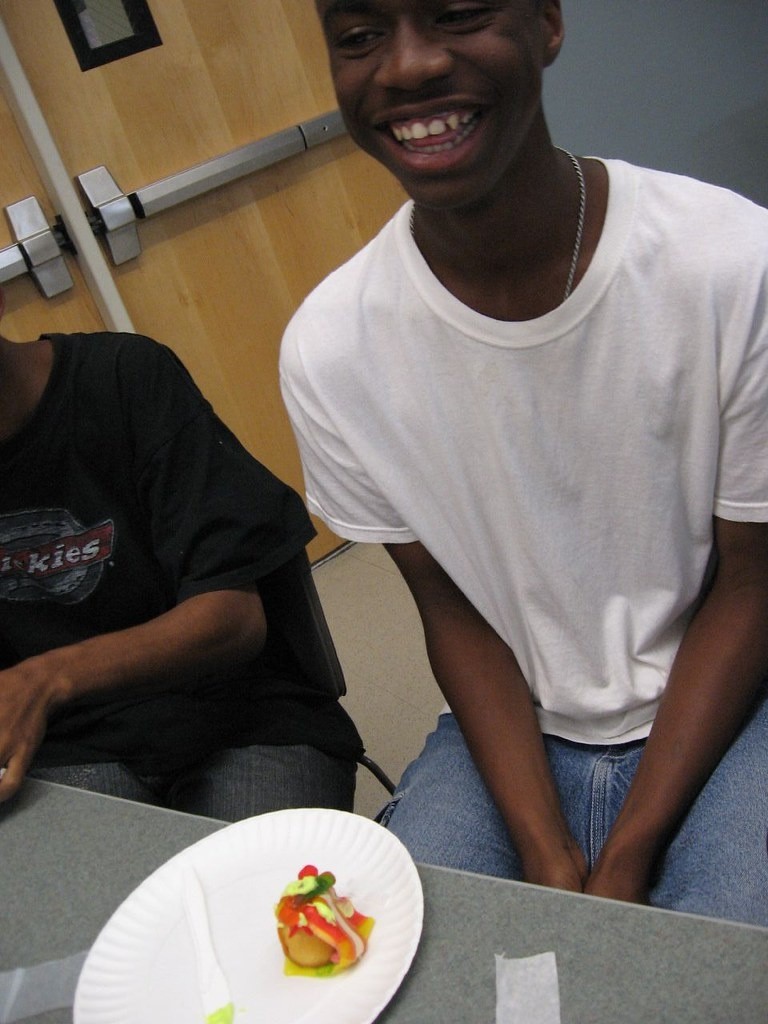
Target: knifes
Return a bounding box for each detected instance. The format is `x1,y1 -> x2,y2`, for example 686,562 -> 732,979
181,868 -> 234,1024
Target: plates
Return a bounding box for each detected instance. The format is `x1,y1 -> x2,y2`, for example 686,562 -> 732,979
72,808 -> 425,1024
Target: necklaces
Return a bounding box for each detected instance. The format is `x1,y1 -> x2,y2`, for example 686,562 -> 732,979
409,145 -> 583,302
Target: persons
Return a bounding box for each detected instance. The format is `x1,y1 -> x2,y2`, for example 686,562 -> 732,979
278,0 -> 768,929
0,328 -> 365,824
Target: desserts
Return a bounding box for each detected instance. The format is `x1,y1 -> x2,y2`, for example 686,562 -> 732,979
274,865 -> 374,978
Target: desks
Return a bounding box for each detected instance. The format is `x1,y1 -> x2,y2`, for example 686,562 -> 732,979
0,766 -> 768,1024
